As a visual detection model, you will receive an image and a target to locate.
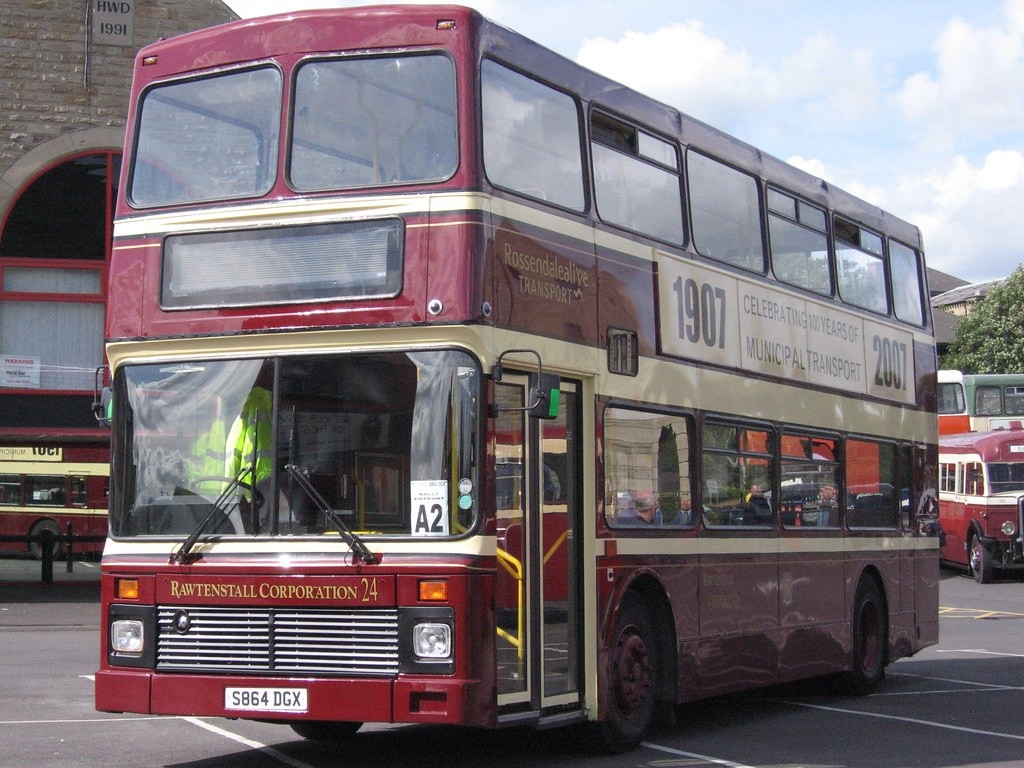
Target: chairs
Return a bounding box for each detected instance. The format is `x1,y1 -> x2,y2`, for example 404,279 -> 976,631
249,472 -> 313,533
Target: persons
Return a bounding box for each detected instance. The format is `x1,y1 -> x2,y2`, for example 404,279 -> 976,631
619,470 -> 839,530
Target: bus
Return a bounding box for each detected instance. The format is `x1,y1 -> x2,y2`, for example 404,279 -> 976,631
938,370 -> 1023,584
0,386 -> 221,559
89,0 -> 943,752
